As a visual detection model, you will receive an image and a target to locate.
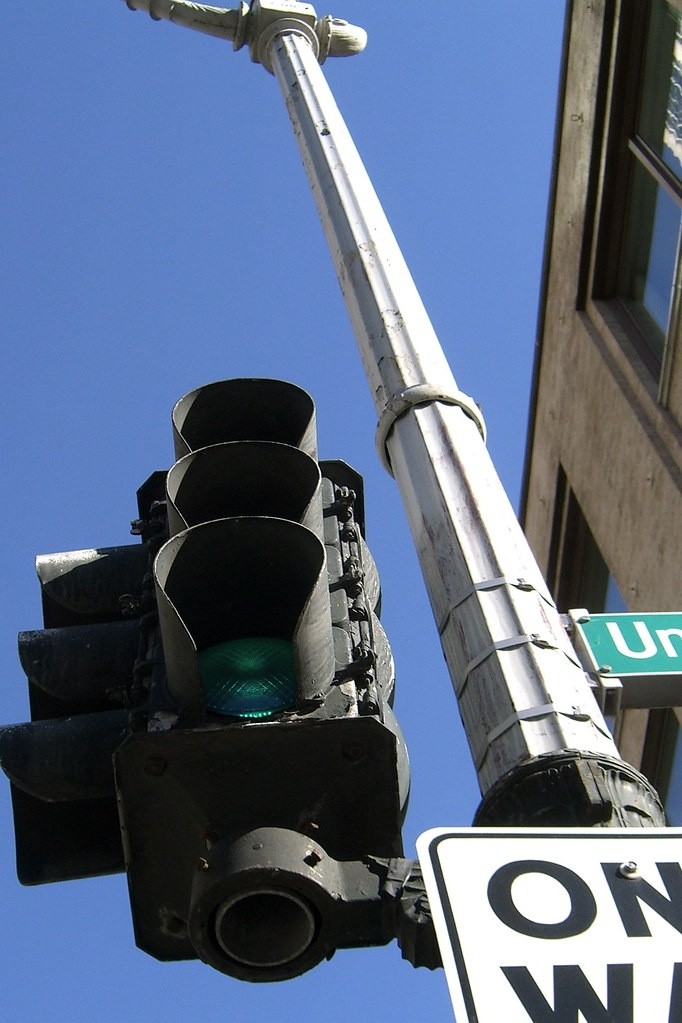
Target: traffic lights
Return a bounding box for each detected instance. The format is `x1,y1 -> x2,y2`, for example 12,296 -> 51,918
0,378 -> 358,886
